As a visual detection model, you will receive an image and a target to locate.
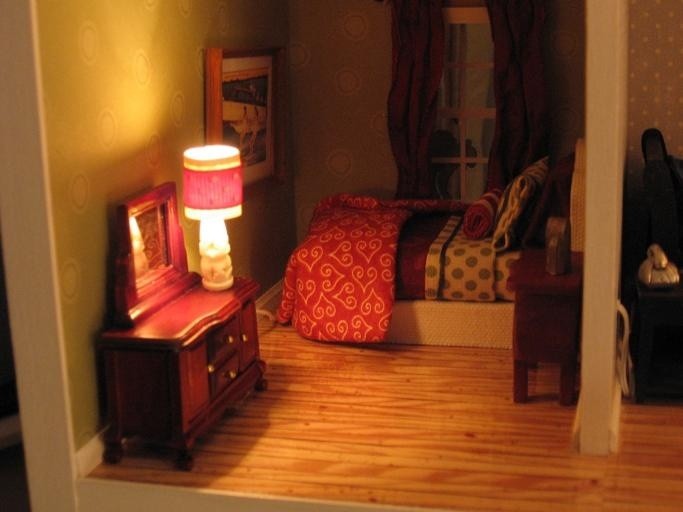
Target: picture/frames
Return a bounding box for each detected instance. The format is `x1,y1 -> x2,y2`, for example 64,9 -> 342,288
202,42 -> 284,189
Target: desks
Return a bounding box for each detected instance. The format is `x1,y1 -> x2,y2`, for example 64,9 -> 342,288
630,289 -> 683,405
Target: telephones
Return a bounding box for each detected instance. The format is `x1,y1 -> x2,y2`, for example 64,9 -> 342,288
638,243 -> 680,288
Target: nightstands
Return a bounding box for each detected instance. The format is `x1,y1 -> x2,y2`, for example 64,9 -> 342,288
508,248 -> 584,405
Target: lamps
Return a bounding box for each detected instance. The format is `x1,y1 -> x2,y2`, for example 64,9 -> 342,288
179,145 -> 243,292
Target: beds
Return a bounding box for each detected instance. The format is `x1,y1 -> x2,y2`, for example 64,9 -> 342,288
298,136 -> 583,351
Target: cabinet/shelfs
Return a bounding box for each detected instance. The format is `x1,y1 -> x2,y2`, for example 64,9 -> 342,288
94,277 -> 271,473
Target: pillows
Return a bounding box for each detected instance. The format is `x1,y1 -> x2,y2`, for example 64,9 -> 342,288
456,154 -> 552,254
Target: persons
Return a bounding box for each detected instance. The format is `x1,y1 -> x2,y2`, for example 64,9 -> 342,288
231,105 -> 265,160
